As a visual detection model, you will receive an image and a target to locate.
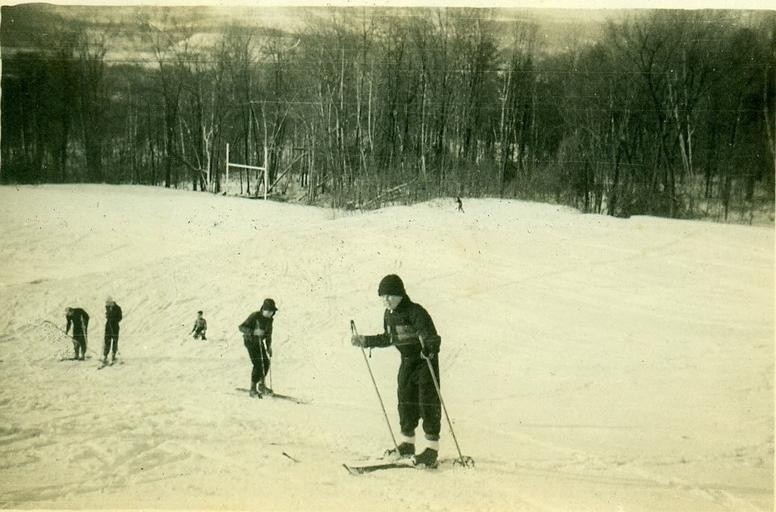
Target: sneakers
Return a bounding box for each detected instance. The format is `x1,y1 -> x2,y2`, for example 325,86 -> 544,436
248,383 -> 273,399
383,442 -> 438,466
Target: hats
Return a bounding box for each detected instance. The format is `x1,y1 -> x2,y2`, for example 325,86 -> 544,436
264,298 -> 278,310
378,274 -> 406,297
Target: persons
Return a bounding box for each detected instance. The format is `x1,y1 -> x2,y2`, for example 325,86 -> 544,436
455,197 -> 465,213
239,298 -> 278,399
351,274 -> 441,466
104,296 -> 122,364
189,311 -> 207,340
64,306 -> 89,360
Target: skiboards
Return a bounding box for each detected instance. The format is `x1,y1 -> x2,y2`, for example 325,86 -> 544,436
227,387 -> 315,404
96,358 -> 126,370
58,356 -> 92,362
282,452 -> 474,475
